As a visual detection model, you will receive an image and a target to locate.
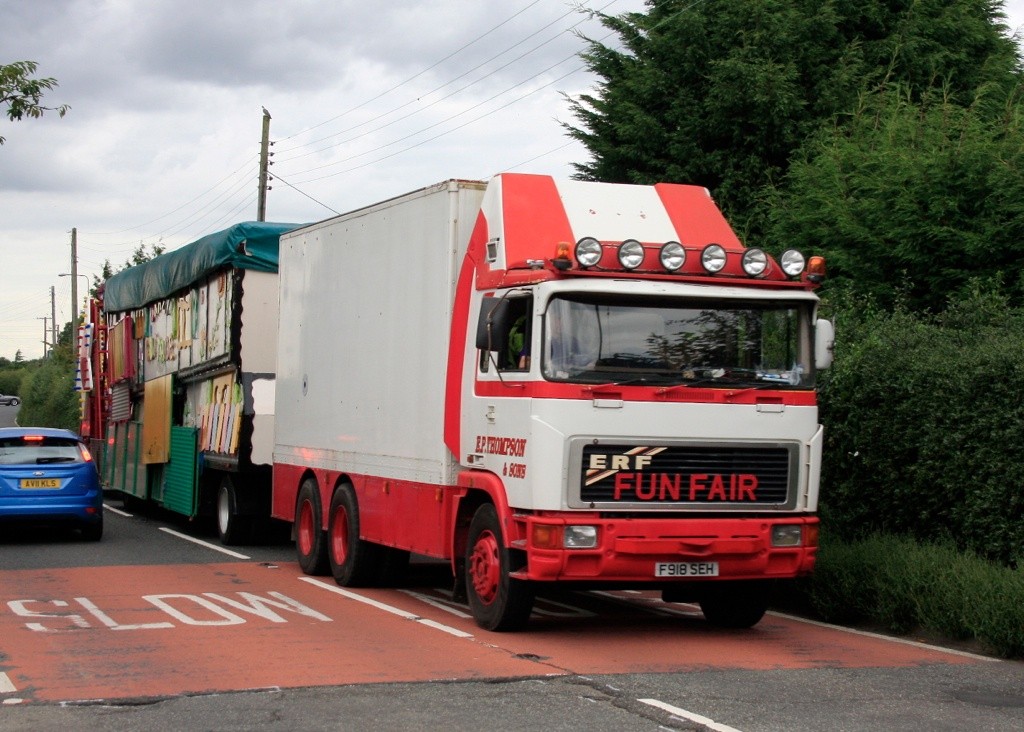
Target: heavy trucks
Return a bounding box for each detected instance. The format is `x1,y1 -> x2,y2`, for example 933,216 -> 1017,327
270,173 -> 839,633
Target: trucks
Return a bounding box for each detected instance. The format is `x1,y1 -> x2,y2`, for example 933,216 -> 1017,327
74,219 -> 314,546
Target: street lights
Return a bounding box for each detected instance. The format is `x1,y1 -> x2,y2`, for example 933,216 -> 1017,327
58,271 -> 90,321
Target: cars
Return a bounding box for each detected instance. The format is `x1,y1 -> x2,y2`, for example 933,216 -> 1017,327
0,394 -> 22,407
0,427 -> 106,542
